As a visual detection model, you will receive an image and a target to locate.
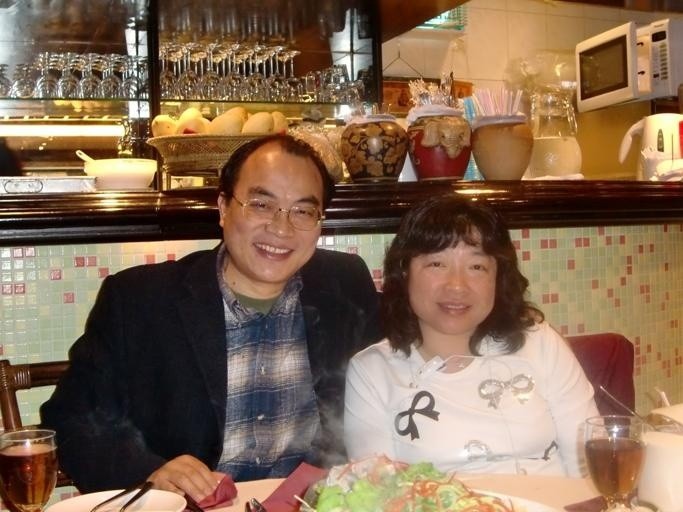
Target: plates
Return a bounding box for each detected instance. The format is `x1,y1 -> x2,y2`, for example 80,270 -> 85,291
466,489 -> 561,512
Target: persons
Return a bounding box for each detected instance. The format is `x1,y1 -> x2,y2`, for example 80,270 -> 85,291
342,191 -> 608,479
37,132 -> 386,506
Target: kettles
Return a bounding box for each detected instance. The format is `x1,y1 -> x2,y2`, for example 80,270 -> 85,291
618,112 -> 683,182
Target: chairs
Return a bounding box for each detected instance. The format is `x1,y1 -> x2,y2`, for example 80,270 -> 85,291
0,359 -> 71,488
562,333 -> 634,438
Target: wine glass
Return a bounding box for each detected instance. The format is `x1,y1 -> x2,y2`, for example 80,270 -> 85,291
156,42 -> 364,104
0,53 -> 149,99
0,429 -> 58,512
584,416 -> 647,512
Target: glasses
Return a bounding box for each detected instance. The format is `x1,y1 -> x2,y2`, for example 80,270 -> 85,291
233,196 -> 325,231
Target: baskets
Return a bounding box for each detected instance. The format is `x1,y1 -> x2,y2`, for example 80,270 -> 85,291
146,133 -> 269,172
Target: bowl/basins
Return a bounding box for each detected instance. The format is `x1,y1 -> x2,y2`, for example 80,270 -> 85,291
41,489 -> 188,512
83,158 -> 158,190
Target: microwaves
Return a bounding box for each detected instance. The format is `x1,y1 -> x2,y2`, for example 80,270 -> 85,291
575,17 -> 683,114
